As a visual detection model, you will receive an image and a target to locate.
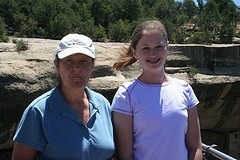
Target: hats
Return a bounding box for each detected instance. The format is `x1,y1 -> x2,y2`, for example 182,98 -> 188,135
55,32 -> 96,60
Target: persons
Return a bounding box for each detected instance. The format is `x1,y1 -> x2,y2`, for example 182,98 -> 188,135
110,20 -> 203,160
11,33 -> 116,160
